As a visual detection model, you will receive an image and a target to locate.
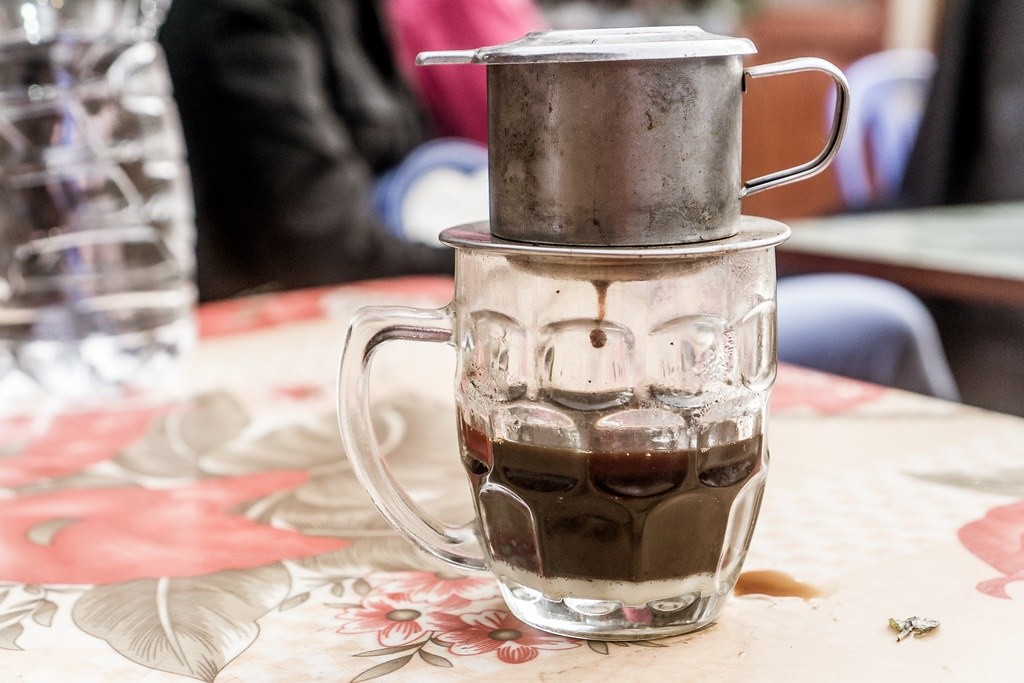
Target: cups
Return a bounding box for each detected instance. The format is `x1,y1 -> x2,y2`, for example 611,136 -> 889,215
410,20 -> 854,246
334,216 -> 797,642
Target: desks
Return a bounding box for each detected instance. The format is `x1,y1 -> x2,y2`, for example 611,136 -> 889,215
768,195 -> 1023,313
2,270 -> 1023,679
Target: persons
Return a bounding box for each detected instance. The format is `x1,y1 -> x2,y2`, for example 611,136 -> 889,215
378,1 -> 567,148
151,1 -> 965,403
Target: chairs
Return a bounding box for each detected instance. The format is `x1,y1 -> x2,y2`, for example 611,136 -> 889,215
826,39 -> 940,214
776,269 -> 972,403
